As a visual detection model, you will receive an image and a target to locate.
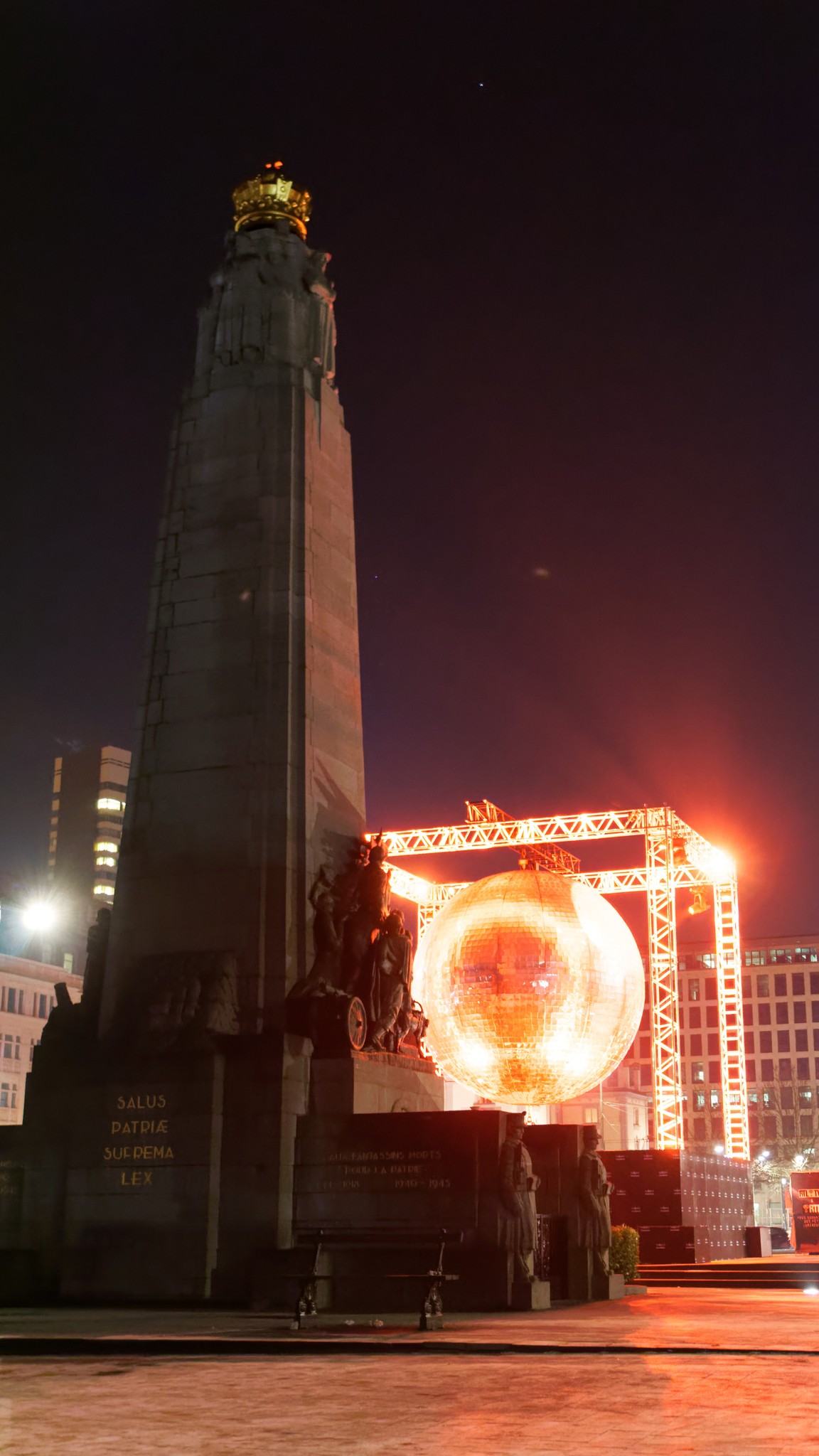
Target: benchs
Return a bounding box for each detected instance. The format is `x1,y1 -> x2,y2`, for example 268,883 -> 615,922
280,1223 -> 461,1336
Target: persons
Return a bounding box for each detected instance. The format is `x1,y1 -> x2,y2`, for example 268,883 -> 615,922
383,909 -> 414,1053
578,1125 -> 614,1277
365,912 -> 411,1051
304,864 -> 340,996
498,1113 -> 540,1282
342,845 -> 390,996
80,908 -> 111,1004
